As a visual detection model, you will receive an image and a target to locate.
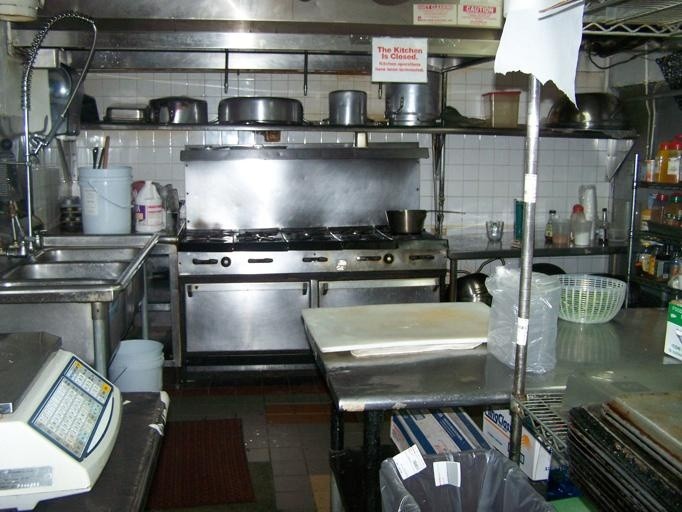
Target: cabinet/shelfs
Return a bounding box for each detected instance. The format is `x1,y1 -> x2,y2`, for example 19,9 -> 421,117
631,178 -> 681,303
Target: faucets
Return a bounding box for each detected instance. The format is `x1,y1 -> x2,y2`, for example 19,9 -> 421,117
7,201 -> 42,258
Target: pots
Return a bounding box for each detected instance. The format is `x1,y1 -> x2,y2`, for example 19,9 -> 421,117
209,96 -> 310,126
385,63 -> 442,127
385,210 -> 466,235
143,96 -> 208,124
328,90 -> 366,126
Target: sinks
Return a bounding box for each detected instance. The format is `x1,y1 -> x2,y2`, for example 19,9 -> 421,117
2,263 -> 128,280
32,249 -> 140,262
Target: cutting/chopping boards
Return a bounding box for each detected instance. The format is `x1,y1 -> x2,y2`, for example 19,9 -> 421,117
300,301 -> 496,352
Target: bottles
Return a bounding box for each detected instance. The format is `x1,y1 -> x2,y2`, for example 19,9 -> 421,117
636,240 -> 682,291
569,204 -> 585,240
639,190 -> 681,234
642,132 -> 681,185
598,208 -> 609,246
544,210 -> 557,242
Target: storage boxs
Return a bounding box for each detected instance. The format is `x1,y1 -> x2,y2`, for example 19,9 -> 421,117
388,399 -> 492,468
481,90 -> 521,130
480,402 -> 552,481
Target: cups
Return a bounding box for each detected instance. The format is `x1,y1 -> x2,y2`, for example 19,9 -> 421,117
167,188 -> 179,212
486,221 -> 504,242
551,219 -> 571,243
574,220 -> 592,246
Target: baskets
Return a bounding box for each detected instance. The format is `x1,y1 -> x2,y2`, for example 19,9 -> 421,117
545,270 -> 628,326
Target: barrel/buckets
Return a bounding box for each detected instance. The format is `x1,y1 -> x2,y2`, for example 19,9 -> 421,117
107,339 -> 165,392
77,165 -> 136,234
135,180 -> 166,233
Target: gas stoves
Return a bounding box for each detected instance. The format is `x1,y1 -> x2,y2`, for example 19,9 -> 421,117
176,225 -> 451,278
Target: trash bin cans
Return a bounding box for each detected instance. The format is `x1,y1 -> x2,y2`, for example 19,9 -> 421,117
378,448 -> 558,512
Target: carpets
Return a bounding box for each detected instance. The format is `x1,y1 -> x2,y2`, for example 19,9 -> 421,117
142,412 -> 258,504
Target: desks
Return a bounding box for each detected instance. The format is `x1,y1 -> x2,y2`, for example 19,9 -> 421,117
446,226 -> 632,308
300,305 -> 682,512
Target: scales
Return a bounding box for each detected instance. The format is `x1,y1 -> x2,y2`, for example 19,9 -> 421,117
0,331 -> 122,512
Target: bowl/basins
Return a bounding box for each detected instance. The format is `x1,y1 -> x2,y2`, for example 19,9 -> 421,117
547,92 -> 633,130
447,271 -> 493,305
